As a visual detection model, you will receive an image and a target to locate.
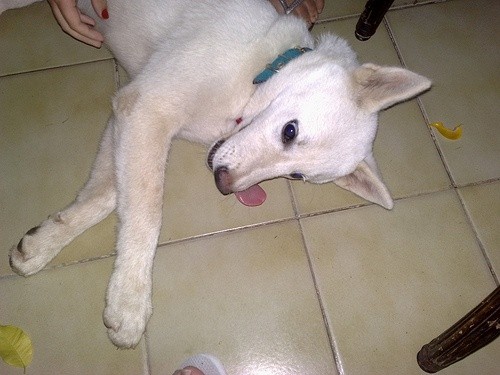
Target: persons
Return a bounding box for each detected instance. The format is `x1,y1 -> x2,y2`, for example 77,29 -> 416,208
47,0 -> 323,48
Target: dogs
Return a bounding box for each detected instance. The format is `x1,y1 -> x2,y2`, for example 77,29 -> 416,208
0,1 -> 431,350
